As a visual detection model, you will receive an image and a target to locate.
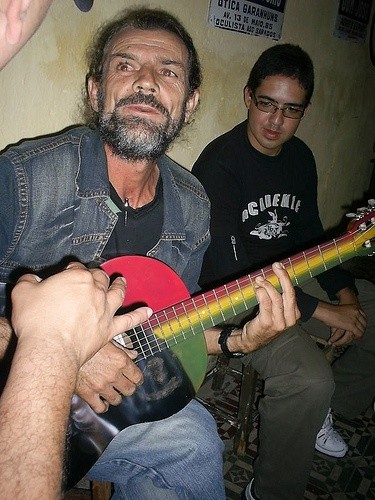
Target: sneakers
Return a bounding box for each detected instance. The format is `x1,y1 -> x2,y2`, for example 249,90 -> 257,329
315,408 -> 348,458
244,477 -> 256,500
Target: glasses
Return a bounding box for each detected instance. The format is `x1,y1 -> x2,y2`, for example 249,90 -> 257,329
251,90 -> 305,119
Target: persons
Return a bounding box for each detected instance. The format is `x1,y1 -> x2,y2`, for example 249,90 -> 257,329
0,1 -> 151,500
0,7 -> 303,499
192,42 -> 367,500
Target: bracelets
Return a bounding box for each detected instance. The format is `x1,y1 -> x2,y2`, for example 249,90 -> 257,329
218,324 -> 247,359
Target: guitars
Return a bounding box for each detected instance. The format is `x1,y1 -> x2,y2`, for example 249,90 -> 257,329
0,198 -> 375,492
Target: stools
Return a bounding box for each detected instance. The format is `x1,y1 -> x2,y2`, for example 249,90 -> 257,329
194,342 -> 334,456
